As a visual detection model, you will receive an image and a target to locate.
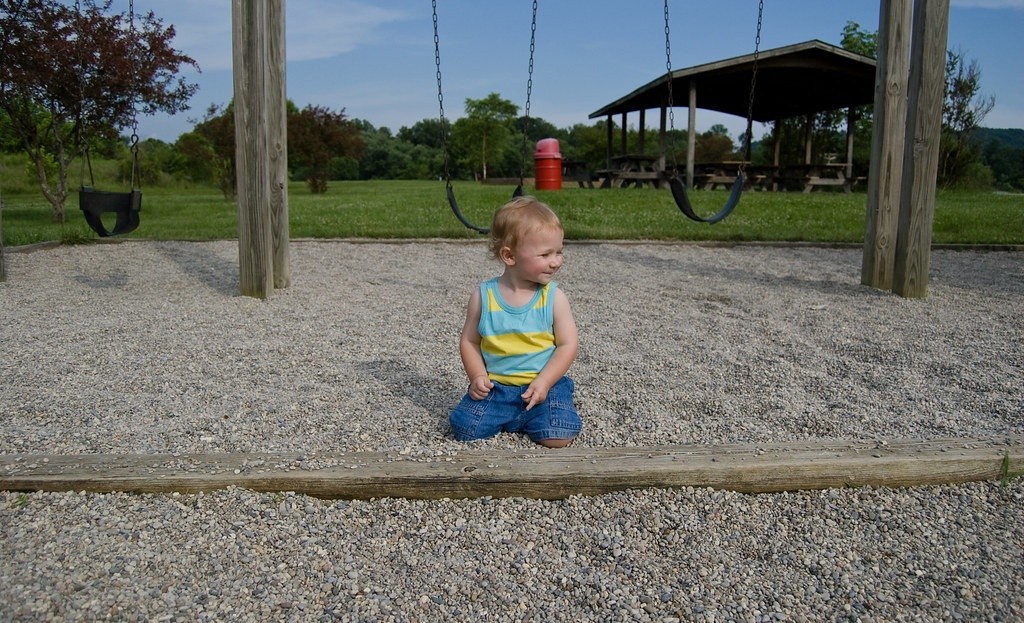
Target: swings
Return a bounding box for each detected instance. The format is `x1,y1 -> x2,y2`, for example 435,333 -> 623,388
663,0 -> 765,224
74,0 -> 145,238
431,0 -> 538,236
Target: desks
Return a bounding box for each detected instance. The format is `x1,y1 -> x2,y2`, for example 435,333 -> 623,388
601,154 -> 662,191
679,160 -> 751,190
744,165 -> 779,192
784,164 -> 854,195
562,161 -> 595,189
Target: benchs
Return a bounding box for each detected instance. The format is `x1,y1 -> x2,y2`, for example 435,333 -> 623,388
590,170 -> 866,194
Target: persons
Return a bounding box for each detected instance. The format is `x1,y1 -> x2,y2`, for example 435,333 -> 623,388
448,195 -> 582,448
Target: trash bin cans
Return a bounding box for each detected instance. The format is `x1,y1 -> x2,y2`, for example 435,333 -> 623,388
533,137 -> 563,190
